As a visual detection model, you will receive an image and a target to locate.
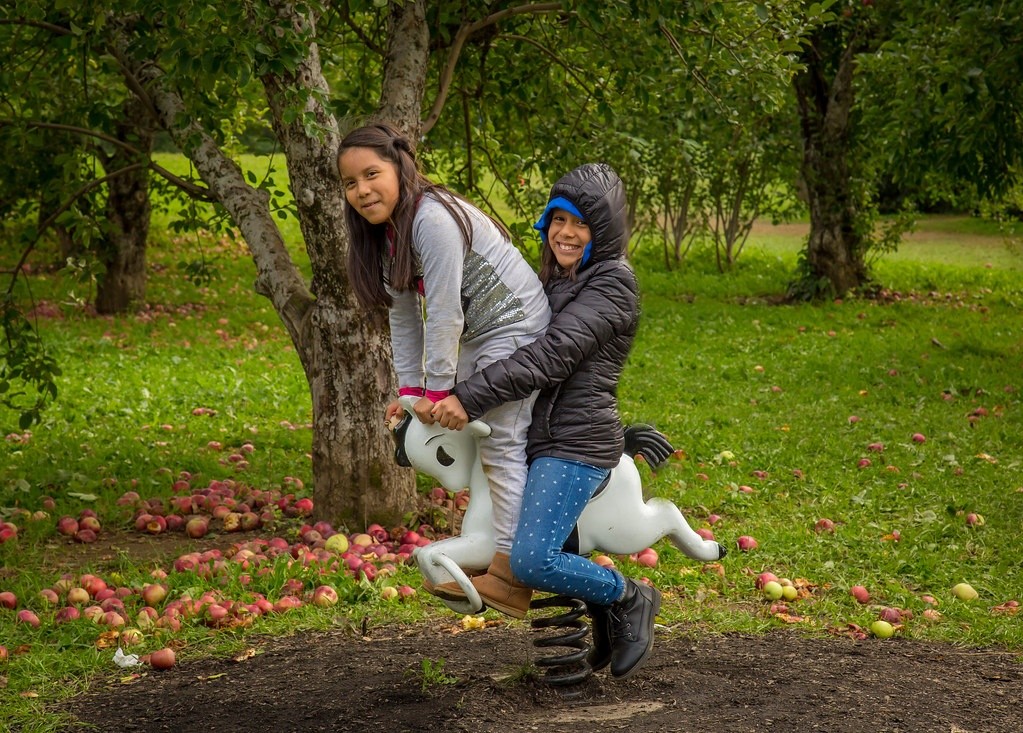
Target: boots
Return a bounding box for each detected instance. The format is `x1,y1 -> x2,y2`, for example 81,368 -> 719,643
434,550 -> 532,620
422,569 -> 487,599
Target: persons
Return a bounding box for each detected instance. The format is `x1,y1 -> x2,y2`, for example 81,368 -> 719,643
429,162 -> 665,681
334,123 -> 552,618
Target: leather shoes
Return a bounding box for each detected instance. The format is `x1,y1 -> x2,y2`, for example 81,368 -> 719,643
584,599 -> 611,671
606,576 -> 661,681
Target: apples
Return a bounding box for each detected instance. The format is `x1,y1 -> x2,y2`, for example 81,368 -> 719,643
0,229 -> 470,672
593,286 -> 1018,641
